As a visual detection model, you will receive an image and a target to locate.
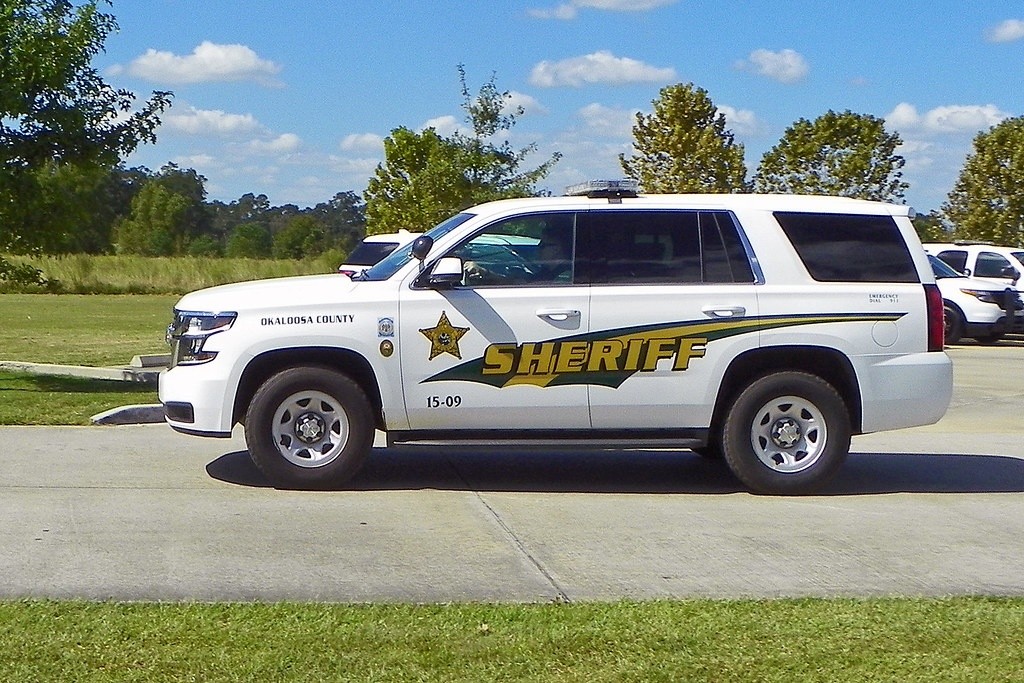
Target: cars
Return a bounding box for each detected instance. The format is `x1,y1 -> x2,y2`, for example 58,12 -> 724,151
923,250 -> 1017,346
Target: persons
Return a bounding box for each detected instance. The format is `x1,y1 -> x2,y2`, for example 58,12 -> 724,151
463,228 -> 572,282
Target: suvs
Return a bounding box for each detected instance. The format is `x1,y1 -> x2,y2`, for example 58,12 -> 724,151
922,239 -> 1024,335
156,178 -> 954,495
338,229 -> 541,284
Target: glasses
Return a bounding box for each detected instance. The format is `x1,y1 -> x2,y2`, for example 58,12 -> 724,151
538,241 -> 561,249
